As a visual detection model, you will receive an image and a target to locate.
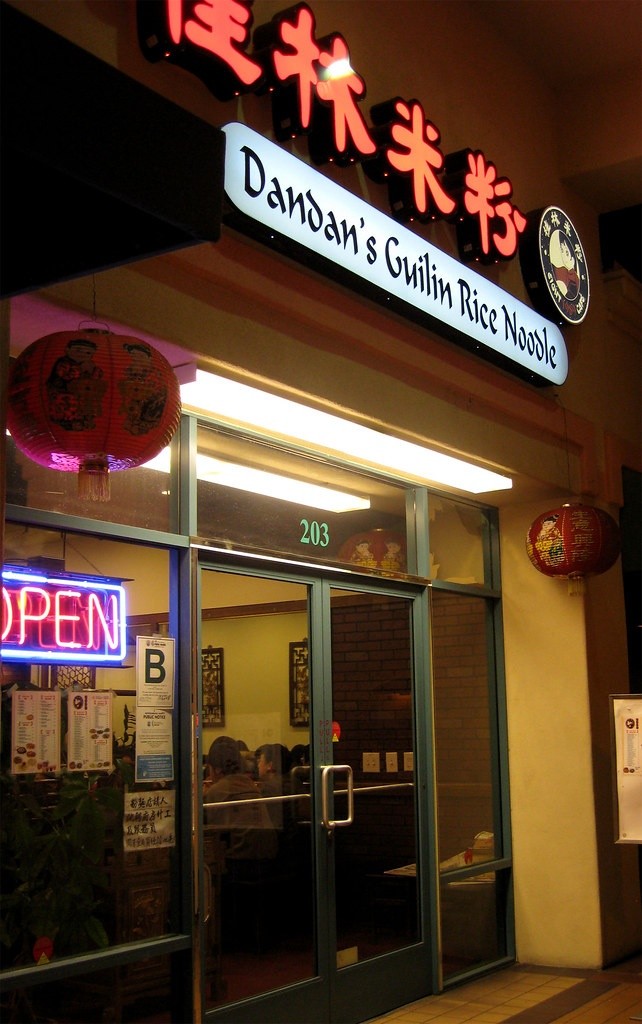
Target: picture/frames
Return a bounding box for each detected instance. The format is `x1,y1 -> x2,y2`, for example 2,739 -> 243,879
202,648 -> 225,728
288,642 -> 309,727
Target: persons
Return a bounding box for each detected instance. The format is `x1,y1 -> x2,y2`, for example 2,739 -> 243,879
203,735 -> 310,863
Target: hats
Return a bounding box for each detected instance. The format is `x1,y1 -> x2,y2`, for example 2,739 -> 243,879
208,736 -> 240,768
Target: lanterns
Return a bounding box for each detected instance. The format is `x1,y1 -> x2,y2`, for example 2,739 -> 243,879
3,329 -> 179,501
525,503 -> 621,595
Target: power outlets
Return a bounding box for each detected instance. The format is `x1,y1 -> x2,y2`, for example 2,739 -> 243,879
404,752 -> 413,771
385,752 -> 397,773
363,752 -> 381,772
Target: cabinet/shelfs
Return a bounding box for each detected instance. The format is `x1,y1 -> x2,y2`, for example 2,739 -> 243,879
61,832 -> 221,1024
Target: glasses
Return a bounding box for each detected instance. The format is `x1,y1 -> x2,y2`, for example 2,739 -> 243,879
257,760 -> 266,764
